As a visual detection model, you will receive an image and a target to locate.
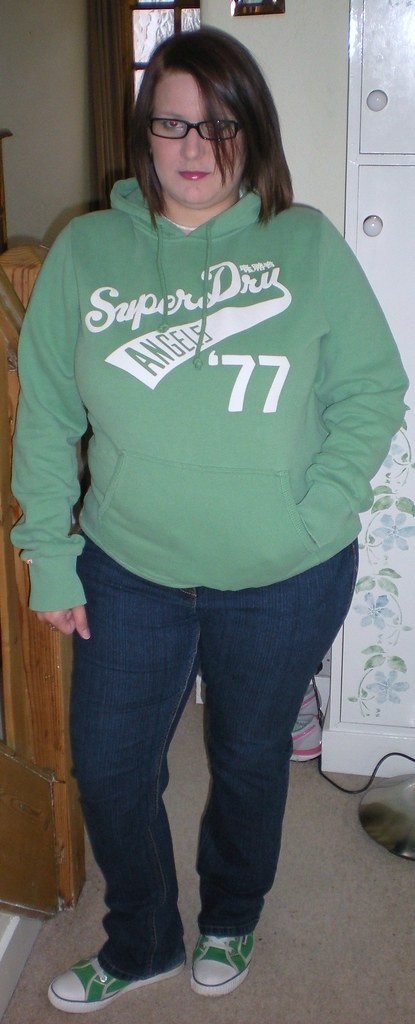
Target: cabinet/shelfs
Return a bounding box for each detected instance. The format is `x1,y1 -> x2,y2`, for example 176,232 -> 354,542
322,0 -> 415,777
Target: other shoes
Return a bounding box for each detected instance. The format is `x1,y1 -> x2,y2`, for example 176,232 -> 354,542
297,684 -> 320,719
289,714 -> 322,762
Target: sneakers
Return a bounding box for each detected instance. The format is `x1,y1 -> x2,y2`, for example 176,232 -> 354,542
190,930 -> 255,997
48,952 -> 186,1014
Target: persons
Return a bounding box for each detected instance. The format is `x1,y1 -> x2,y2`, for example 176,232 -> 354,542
10,26 -> 408,1013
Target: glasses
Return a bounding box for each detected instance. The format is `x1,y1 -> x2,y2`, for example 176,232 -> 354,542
147,117 -> 246,140
